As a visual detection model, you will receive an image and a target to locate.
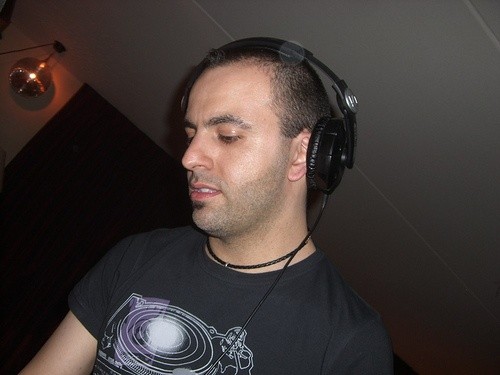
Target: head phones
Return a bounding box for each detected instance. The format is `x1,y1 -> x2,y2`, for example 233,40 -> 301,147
179,35 -> 359,194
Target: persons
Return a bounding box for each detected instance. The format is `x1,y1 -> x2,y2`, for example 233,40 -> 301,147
20,37 -> 402,375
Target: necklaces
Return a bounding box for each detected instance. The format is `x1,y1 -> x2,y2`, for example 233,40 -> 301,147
204,233 -> 311,269
